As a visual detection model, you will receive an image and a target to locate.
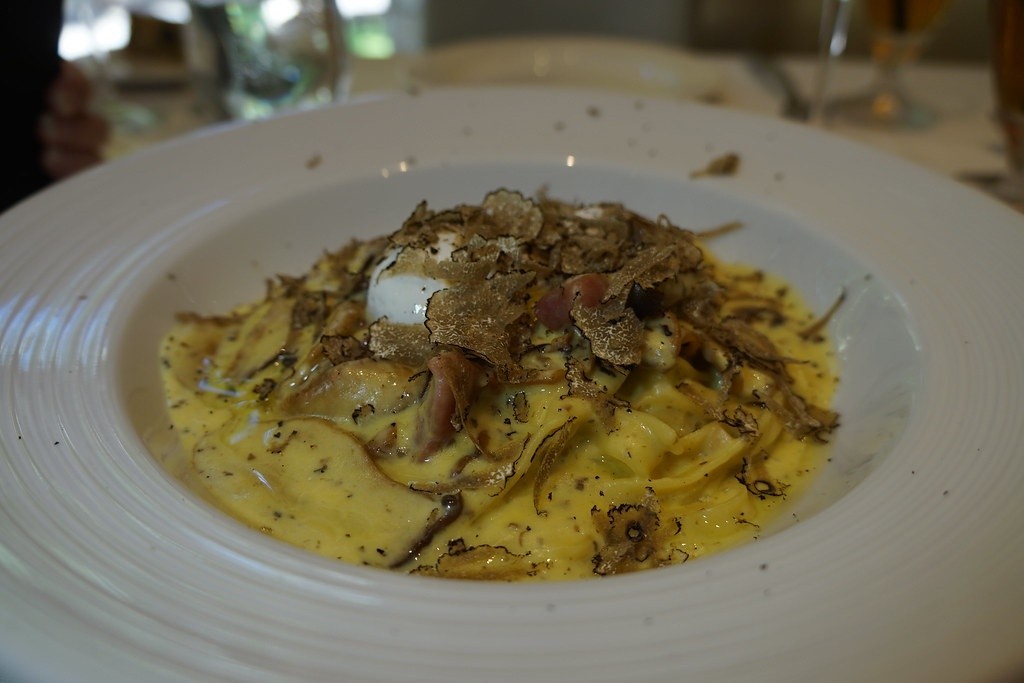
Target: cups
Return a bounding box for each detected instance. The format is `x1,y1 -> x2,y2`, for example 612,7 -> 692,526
188,0 -> 344,120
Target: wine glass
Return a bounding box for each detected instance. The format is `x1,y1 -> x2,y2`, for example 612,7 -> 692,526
828,0 -> 953,129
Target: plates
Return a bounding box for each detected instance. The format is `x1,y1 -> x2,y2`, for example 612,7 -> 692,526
0,84 -> 1024,683
